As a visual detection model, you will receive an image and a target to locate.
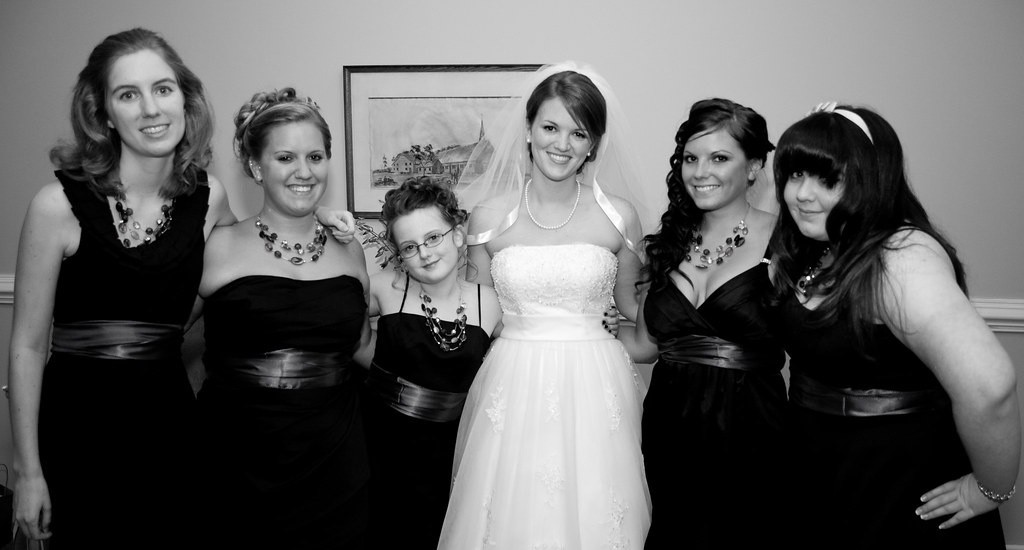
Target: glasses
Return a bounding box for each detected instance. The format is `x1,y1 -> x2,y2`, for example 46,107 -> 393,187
397,224 -> 460,260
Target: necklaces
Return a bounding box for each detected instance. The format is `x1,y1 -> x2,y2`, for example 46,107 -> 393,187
525,178 -> 581,230
796,247 -> 830,293
420,288 -> 466,351
256,213 -> 327,264
683,205 -> 752,269
113,181 -> 176,249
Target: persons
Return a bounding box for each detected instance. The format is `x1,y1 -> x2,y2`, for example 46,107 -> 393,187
769,105 -> 1022,550
10,27 -> 240,550
605,97 -> 820,550
191,90 -> 379,550
436,62 -> 653,550
342,178 -> 621,550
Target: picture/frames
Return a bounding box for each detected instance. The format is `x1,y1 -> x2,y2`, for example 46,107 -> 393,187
343,64 -> 553,220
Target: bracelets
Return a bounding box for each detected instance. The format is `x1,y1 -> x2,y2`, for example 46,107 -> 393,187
978,482 -> 1015,502
